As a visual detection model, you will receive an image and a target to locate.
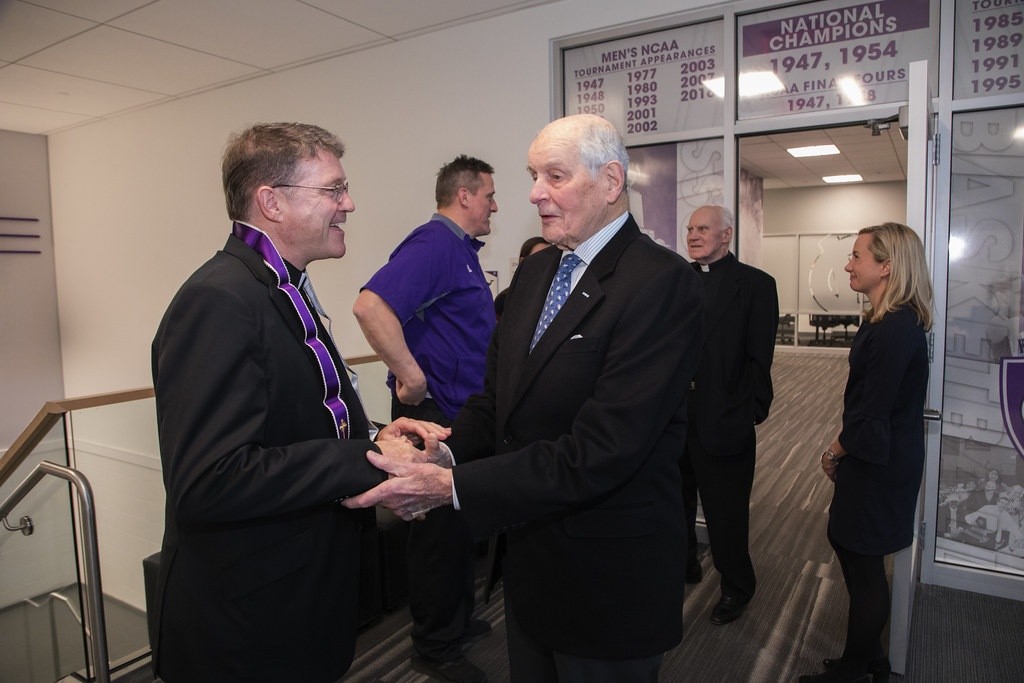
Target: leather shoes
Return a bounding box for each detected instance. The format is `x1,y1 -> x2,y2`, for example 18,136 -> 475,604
686,562 -> 704,583
709,573 -> 756,625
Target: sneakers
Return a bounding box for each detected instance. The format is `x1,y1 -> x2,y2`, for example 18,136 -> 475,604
460,619 -> 492,643
409,650 -> 488,683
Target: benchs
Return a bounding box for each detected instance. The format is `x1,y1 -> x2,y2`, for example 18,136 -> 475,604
144,505 -> 414,671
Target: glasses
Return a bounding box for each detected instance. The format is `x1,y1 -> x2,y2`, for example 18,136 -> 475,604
272,182 -> 348,204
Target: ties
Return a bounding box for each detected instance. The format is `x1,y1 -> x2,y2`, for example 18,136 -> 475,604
525,252 -> 585,358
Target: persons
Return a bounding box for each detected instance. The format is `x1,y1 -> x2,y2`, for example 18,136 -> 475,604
937,469 -> 1024,559
682,203 -> 780,622
351,156 -> 498,683
495,236 -> 552,318
342,114 -> 709,682
149,121 -> 455,683
798,223 -> 931,683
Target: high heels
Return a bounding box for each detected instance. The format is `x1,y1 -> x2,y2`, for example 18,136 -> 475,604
798,660 -> 870,683
822,656 -> 891,683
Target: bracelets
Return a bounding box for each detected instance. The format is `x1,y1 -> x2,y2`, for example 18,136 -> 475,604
828,445 -> 838,462
820,451 -> 838,469
